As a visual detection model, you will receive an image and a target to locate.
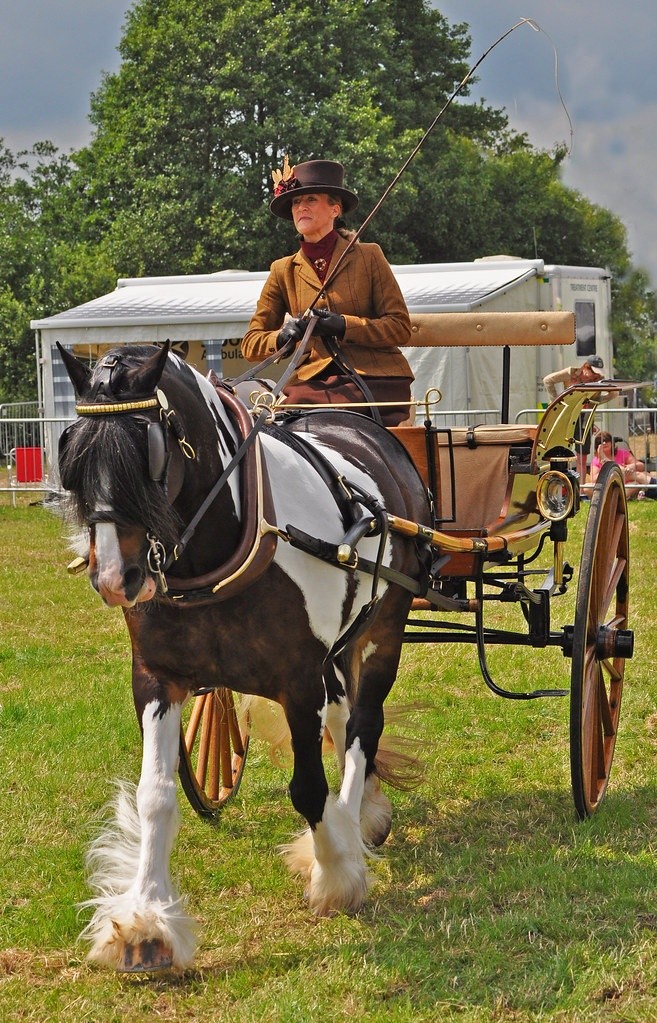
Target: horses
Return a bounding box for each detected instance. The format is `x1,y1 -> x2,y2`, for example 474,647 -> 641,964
50,340 -> 438,974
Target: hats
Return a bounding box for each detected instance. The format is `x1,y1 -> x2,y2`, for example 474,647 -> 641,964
269,160 -> 360,219
587,355 -> 605,376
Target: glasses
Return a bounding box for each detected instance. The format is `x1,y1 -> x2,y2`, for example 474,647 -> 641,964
598,437 -> 611,444
591,369 -> 593,373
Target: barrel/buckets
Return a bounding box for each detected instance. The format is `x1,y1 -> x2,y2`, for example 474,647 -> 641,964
15,446 -> 43,483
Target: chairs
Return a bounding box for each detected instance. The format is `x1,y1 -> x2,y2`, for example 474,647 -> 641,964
7,447 -> 49,506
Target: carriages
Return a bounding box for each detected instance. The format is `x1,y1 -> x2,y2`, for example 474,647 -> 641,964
53,311 -> 639,974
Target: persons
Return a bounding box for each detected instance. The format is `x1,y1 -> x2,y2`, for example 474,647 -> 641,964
243,162 -> 410,427
543,354 -> 656,500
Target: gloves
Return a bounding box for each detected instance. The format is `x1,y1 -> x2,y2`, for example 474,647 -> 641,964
278,318 -> 307,354
307,309 -> 345,338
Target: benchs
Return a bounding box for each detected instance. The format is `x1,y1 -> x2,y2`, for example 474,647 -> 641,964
405,311 -> 576,476
